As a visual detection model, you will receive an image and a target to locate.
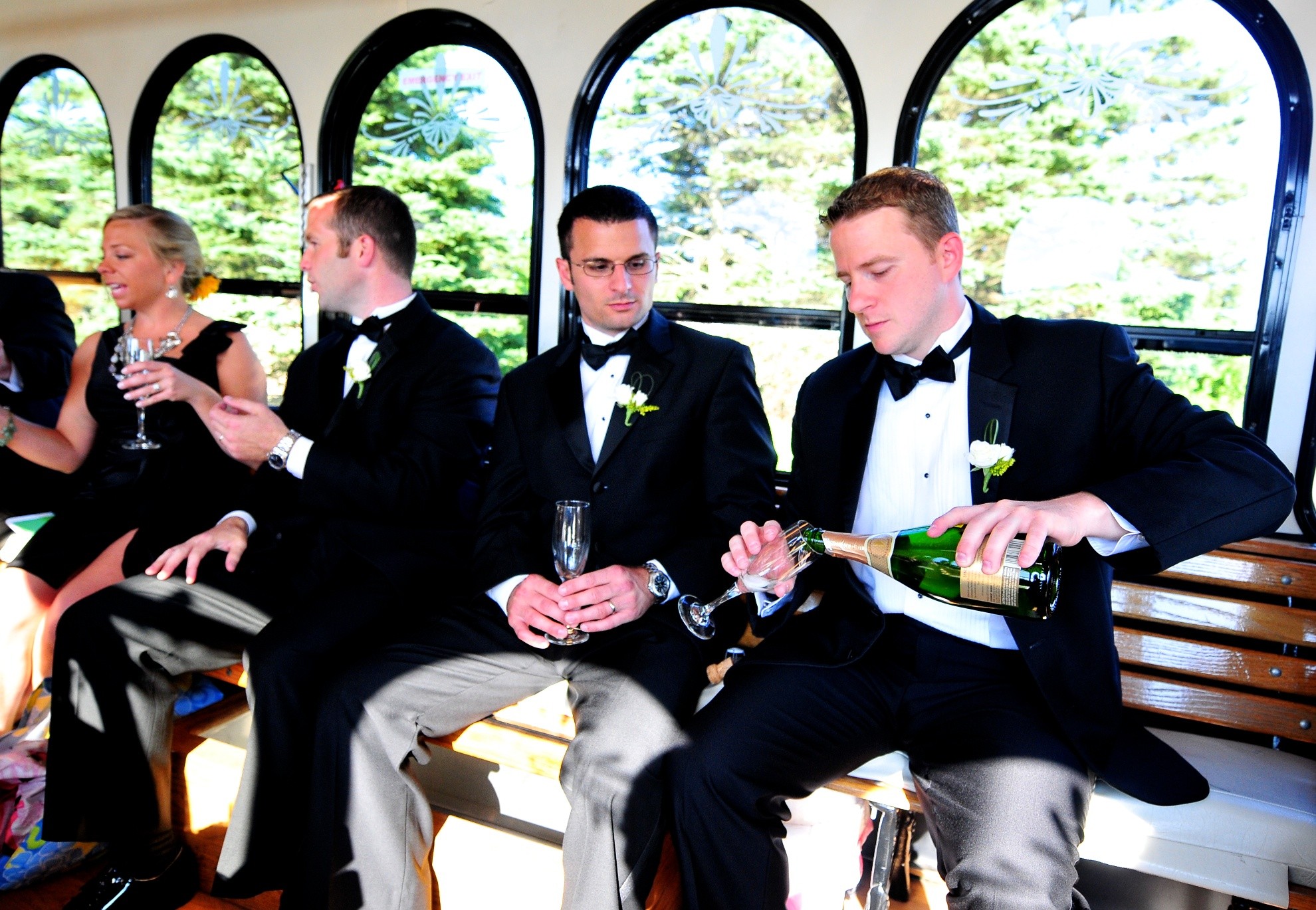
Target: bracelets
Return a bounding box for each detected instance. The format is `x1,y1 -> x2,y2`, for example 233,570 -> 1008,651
0,404 -> 16,447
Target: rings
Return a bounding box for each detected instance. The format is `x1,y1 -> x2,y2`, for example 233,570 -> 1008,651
154,382 -> 161,394
607,599 -> 617,614
748,554 -> 757,563
218,433 -> 224,442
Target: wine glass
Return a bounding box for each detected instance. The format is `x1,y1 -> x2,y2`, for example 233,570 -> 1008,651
116,333 -> 162,451
540,497 -> 595,643
676,520 -> 827,641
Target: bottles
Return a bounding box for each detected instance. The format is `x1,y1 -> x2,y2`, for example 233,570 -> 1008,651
804,522 -> 1062,622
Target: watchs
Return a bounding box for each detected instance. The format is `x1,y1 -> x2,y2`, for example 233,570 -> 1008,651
268,427 -> 302,471
641,563 -> 670,605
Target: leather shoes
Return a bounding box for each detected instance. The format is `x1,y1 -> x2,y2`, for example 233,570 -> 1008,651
61,846 -> 200,910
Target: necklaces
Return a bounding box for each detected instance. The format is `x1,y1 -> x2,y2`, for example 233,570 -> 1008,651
108,304 -> 192,388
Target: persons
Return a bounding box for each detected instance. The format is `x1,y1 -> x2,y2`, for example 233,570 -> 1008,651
0,205 -> 270,746
40,186 -> 501,910
666,164 -> 1295,908
324,186 -> 777,910
1,271 -> 78,544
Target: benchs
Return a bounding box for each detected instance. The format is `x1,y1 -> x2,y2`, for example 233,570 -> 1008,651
193,460 -> 1316,910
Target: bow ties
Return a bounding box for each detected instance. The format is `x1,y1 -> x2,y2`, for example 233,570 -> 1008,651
576,323 -> 647,371
331,309 -> 404,343
878,325 -> 972,402
